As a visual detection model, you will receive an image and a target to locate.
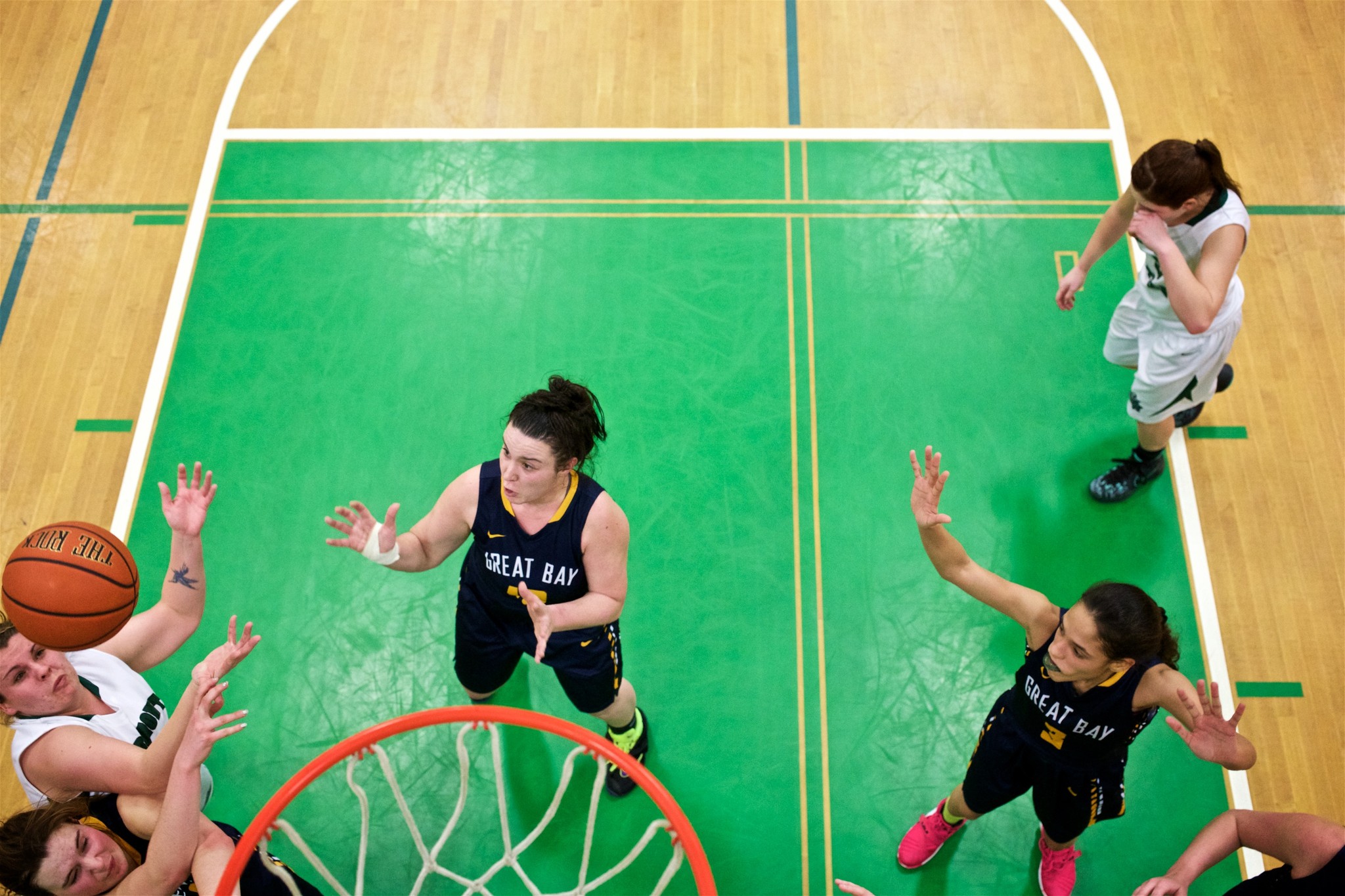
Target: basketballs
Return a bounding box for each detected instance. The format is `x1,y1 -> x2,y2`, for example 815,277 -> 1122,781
3,520 -> 139,651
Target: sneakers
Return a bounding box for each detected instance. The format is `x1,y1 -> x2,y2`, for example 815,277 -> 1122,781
1173,363 -> 1233,428
897,796 -> 968,869
601,707 -> 647,797
1090,453 -> 1165,502
1037,821 -> 1082,896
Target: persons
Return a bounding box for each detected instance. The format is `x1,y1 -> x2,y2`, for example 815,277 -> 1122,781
0,678 -> 324,896
0,462 -> 260,814
325,372 -> 649,796
897,445 -> 1256,896
1056,138 -> 1251,503
1131,808 -> 1345,896
